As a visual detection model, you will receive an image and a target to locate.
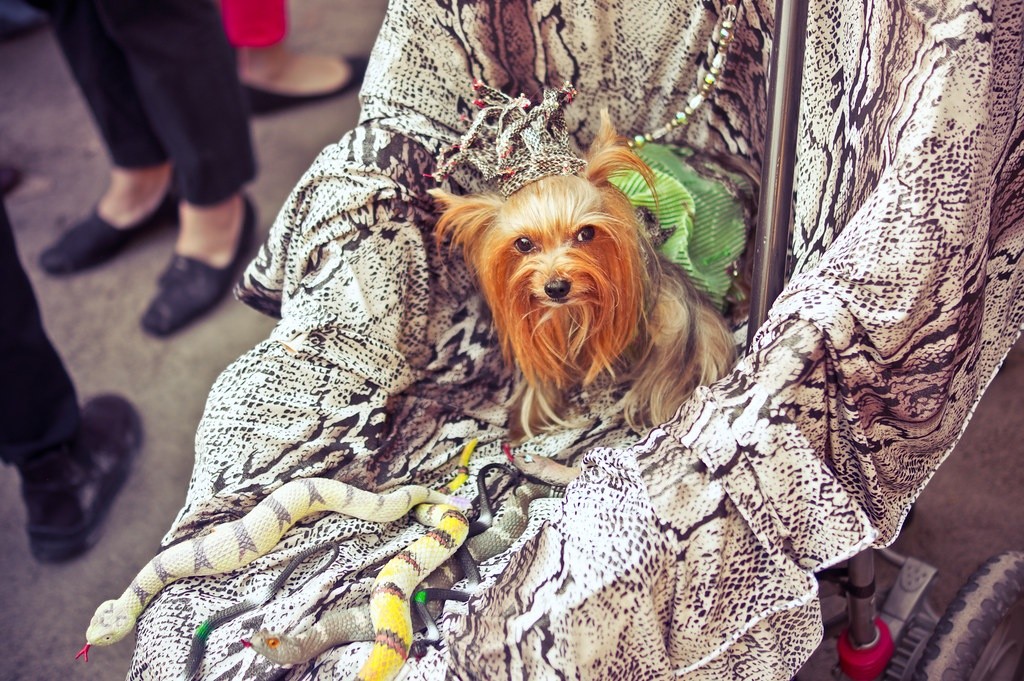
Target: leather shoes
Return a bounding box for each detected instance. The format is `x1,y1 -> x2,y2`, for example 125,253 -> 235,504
141,190 -> 256,337
20,391 -> 144,563
38,176 -> 178,277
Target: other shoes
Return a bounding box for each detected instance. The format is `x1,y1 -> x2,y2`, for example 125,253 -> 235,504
235,49 -> 370,116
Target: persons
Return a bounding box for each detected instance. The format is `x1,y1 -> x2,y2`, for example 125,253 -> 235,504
222,0 -> 375,114
31,0 -> 262,339
1,190 -> 149,563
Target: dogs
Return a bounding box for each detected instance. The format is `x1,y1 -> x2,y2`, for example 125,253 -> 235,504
424,106 -> 740,450
